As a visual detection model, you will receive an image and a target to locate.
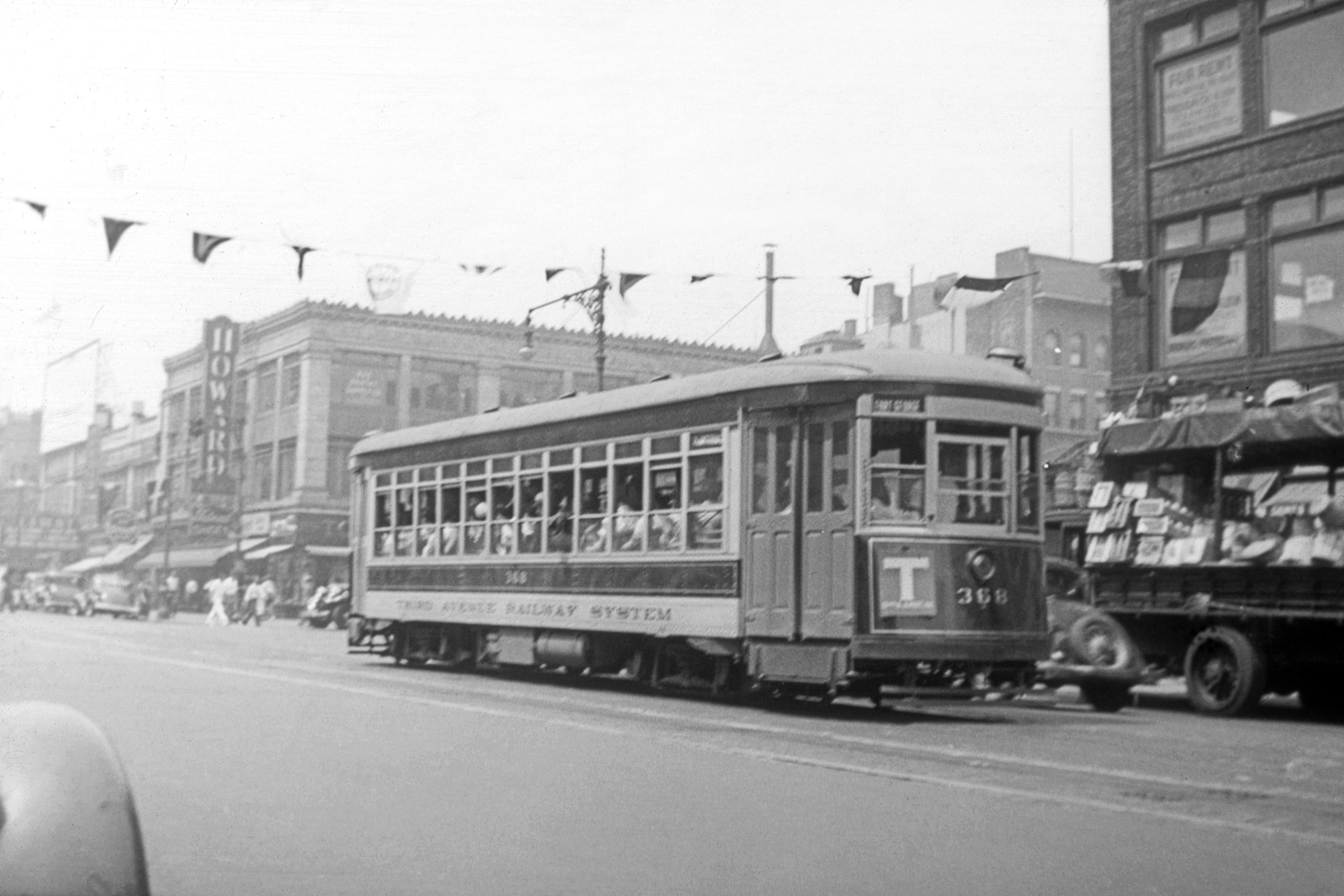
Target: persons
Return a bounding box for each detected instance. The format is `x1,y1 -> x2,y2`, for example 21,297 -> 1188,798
374,453 -> 976,553
161,569 -> 349,629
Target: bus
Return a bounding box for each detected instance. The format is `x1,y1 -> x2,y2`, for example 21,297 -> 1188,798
342,348 -> 1052,710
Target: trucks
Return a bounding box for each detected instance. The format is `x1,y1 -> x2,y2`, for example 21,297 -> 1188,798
1084,560 -> 1343,718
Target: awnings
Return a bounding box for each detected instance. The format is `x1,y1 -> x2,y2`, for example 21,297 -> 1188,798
132,549 -> 230,570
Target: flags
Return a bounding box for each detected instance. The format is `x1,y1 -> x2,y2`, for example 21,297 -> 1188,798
940,272 -> 1039,311
1169,247 -> 1231,337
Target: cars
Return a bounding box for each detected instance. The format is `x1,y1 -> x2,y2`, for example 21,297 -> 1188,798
70,573 -> 142,617
1038,559 -> 1158,713
13,571 -> 80,610
